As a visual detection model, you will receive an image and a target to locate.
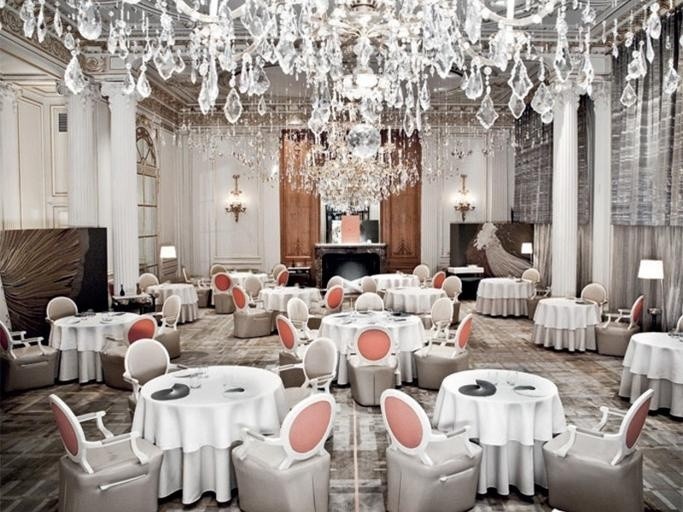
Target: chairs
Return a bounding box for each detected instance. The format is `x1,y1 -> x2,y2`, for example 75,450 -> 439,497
544,388 -> 655,510
230,393 -> 335,510
48,394 -> 162,510
380,388 -> 484,510
0,322 -> 57,390
45,296 -> 79,324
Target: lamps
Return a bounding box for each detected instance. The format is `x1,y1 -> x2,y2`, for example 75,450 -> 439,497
160,246 -> 178,281
521,242 -> 534,278
226,173 -> 248,222
637,259 -> 665,315
453,173 -> 475,221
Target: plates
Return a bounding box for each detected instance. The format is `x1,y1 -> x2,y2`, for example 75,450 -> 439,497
514,384 -> 546,398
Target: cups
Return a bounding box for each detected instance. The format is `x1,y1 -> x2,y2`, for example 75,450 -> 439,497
181,359 -> 235,391
486,366 -> 519,388
